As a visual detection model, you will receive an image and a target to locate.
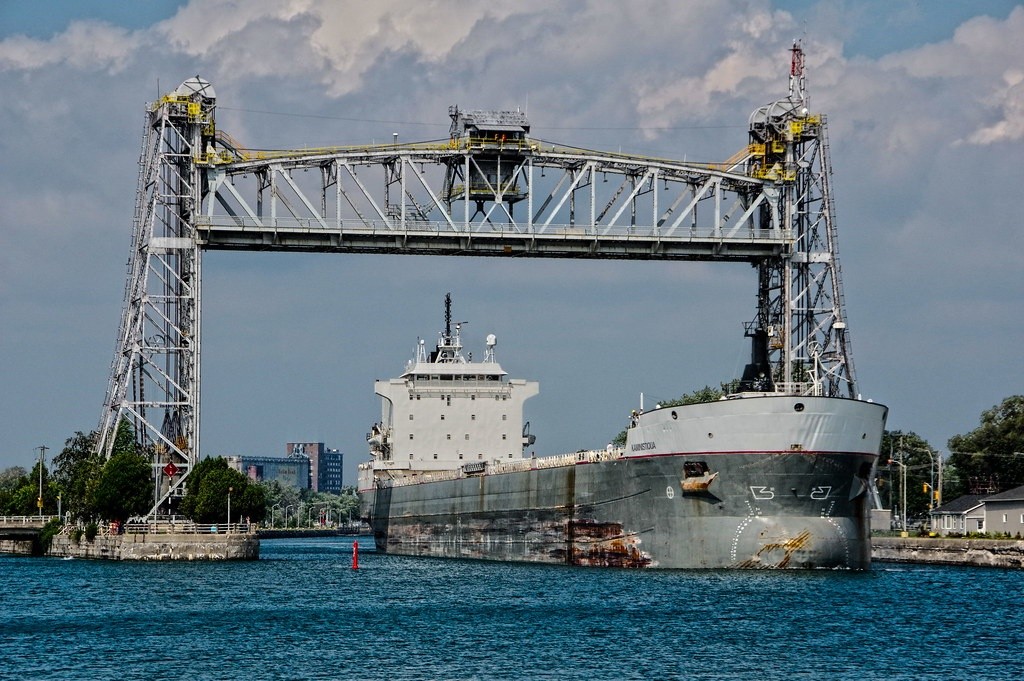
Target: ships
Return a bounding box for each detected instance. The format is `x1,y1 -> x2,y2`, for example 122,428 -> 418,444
358,292 -> 891,571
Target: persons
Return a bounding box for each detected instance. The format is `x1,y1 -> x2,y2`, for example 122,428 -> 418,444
139,520 -> 144,534
128,519 -> 135,533
260,520 -> 264,528
145,520 -> 148,534
372,423 -> 380,435
632,410 -> 638,427
135,520 -> 139,533
118,522 -> 124,535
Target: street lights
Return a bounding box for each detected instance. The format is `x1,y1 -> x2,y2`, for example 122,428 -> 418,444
272,504 -> 345,529
888,460 -> 909,538
37,445 -> 50,516
924,451 -> 934,511
228,487 -> 233,531
